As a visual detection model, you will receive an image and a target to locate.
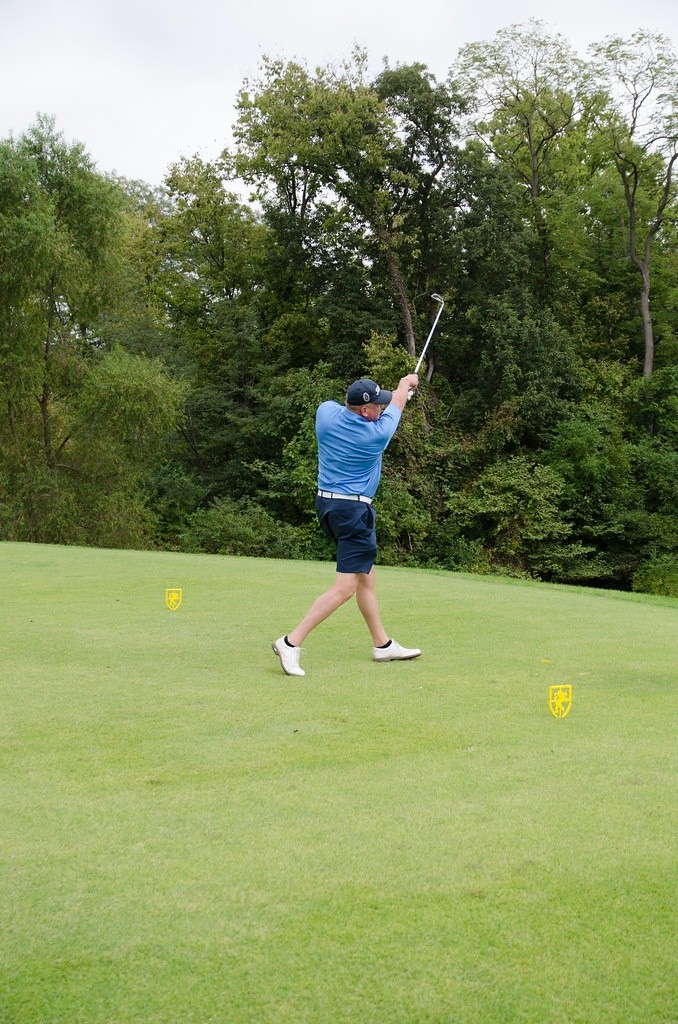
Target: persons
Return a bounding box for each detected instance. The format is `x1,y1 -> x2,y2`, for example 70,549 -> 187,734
273,374 -> 422,676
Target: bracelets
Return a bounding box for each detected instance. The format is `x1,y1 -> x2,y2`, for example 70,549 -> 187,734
405,378 -> 411,384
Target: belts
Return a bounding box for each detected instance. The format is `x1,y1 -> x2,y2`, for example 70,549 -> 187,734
317,489 -> 373,505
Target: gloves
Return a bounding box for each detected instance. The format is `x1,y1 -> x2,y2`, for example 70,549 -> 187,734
407,387 -> 415,402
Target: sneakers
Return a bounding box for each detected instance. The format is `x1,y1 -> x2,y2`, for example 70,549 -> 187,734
271,635 -> 305,676
373,639 -> 421,662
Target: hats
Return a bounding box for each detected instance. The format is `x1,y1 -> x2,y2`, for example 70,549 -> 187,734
346,379 -> 392,406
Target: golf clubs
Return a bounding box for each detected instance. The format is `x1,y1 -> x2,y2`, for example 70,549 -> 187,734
413,293 -> 444,373
